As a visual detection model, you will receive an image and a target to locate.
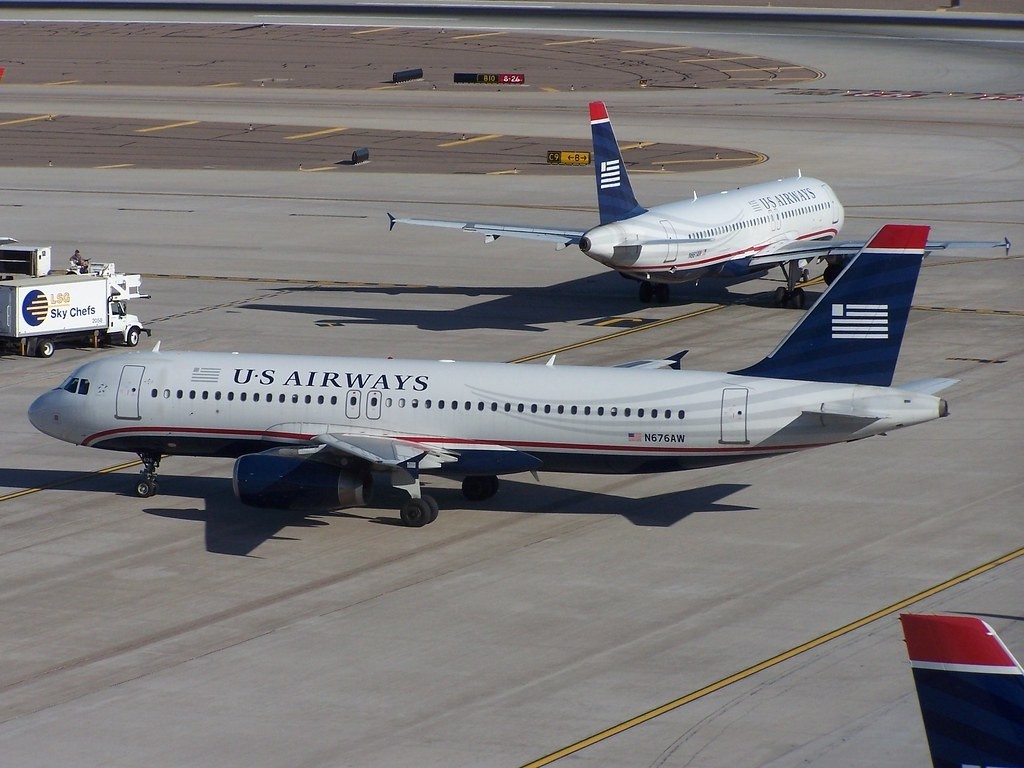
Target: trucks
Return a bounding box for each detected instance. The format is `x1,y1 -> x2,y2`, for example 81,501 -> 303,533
0,273 -> 151,358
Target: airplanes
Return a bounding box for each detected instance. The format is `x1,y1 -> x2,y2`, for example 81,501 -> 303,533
900,613 -> 1024,768
28,224 -> 962,527
387,101 -> 1014,309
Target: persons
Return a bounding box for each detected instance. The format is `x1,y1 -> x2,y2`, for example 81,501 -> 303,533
69,250 -> 88,274
